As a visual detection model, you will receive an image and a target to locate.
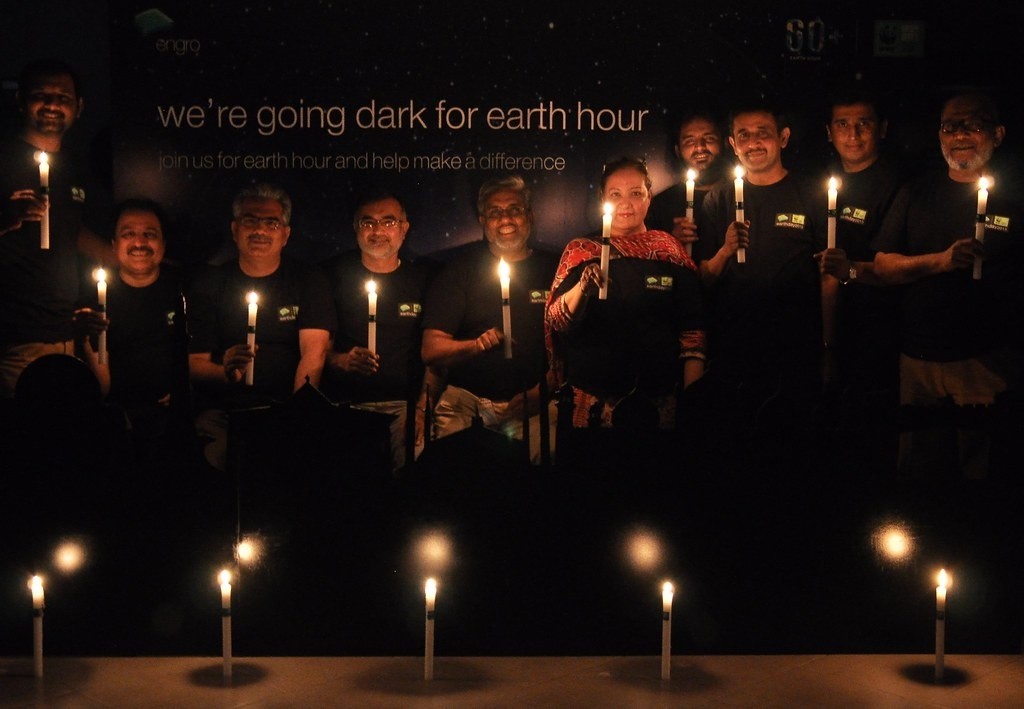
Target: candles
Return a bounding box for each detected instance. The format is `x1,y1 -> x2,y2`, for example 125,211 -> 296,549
935,570 -> 947,680
32,576 -> 44,677
220,570 -> 232,677
498,257 -> 512,359
425,578 -> 436,681
685,169 -> 695,255
828,177 -> 837,248
366,280 -> 377,353
973,177 -> 988,279
599,203 -> 612,299
245,292 -> 258,385
97,269 -> 107,363
39,151 -> 50,249
662,583 -> 673,680
734,166 -> 745,263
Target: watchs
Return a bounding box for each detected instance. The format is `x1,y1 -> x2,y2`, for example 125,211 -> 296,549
840,263 -> 857,285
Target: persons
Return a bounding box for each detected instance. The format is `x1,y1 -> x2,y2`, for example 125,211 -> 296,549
420,174 -> 558,458
646,113 -> 722,242
870,93 -> 1024,478
68,198 -> 193,406
0,58 -> 112,388
177,174 -> 338,470
693,98 -> 840,381
543,155 -> 705,438
325,190 -> 441,470
817,84 -> 929,319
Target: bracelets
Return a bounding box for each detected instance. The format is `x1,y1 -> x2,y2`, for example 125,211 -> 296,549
580,281 -> 592,296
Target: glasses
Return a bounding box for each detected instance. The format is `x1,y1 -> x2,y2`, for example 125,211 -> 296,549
939,117 -> 986,134
480,204 -> 531,218
233,216 -> 285,232
351,216 -> 405,228
